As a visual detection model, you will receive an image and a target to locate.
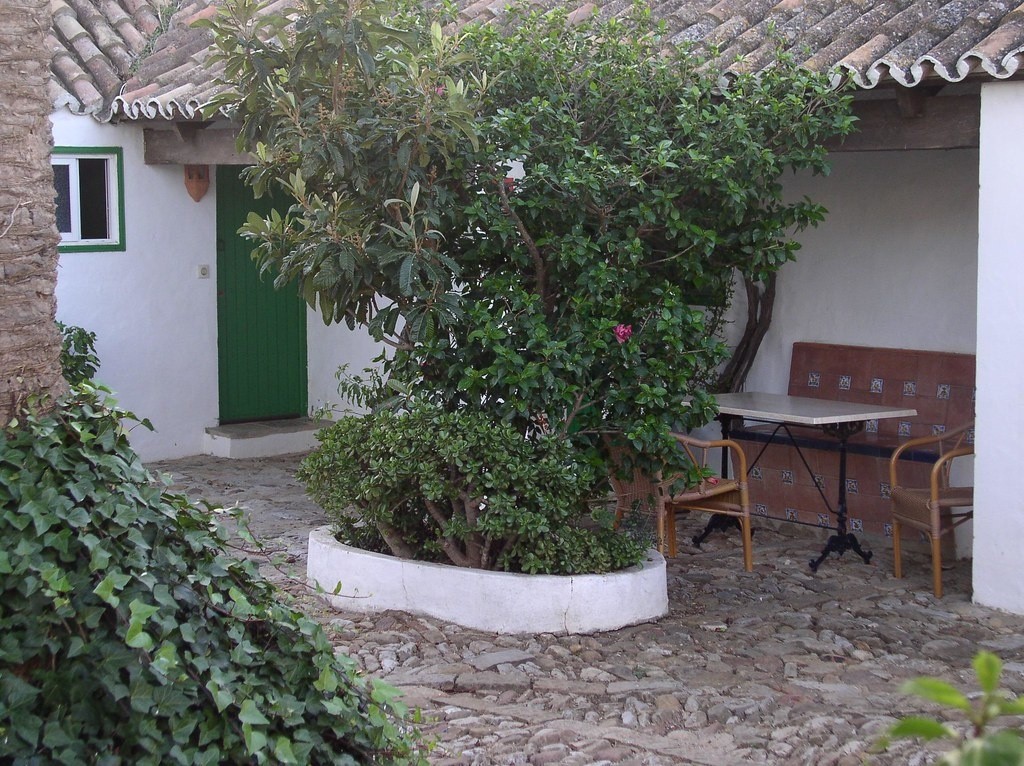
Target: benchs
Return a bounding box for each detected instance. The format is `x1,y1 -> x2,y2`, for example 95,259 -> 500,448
730,342 -> 976,560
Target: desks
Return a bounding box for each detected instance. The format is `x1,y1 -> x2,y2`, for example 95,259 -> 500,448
681,391 -> 918,574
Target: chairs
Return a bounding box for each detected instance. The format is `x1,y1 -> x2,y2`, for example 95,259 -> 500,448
888,421 -> 975,599
578,429 -> 754,574
